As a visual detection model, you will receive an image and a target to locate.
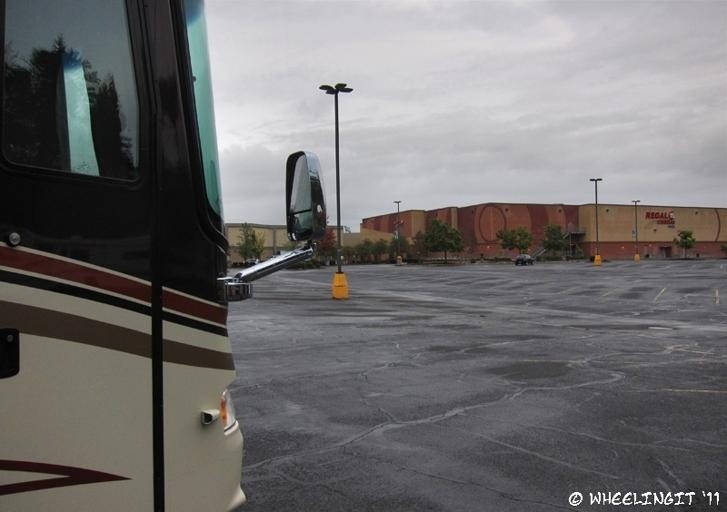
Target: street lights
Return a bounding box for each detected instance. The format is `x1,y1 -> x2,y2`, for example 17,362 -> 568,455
393,200 -> 403,266
589,178 -> 602,265
631,200 -> 640,261
317,82 -> 354,299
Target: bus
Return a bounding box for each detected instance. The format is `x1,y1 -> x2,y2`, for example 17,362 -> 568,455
0,0 -> 326,511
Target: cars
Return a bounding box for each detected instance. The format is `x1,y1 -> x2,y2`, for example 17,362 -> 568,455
246,258 -> 258,264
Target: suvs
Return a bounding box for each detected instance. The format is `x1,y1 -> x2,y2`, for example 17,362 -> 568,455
515,254 -> 534,265
